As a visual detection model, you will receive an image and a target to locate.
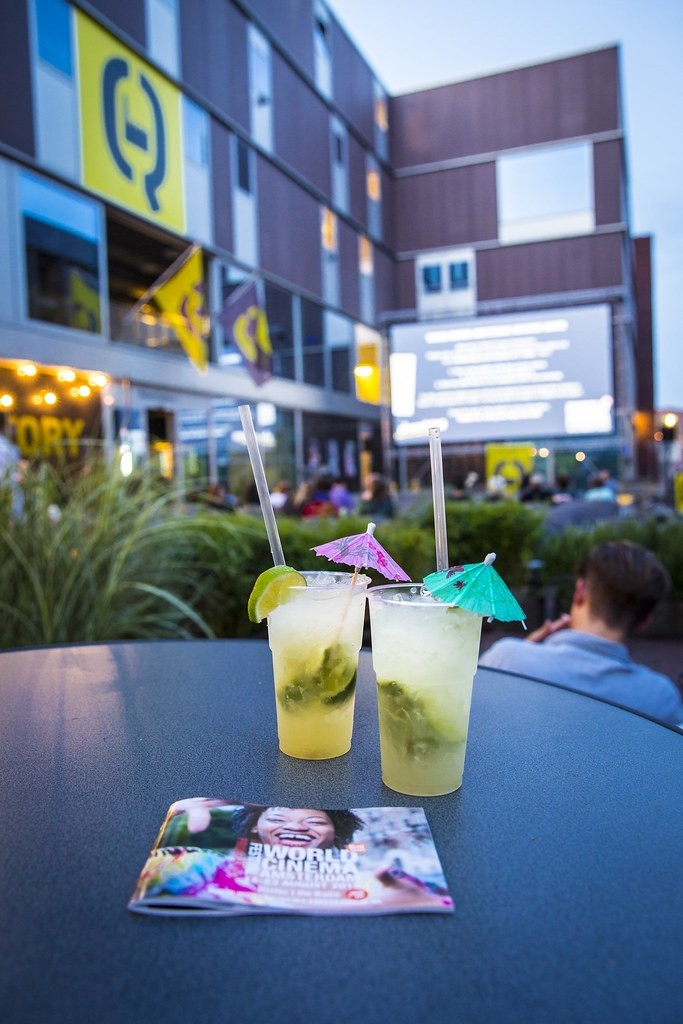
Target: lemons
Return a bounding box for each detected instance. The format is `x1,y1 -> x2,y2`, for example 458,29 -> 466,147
248,564 -> 308,623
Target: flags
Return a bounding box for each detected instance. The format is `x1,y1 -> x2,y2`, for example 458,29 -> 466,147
158,250 -> 209,372
223,281 -> 275,385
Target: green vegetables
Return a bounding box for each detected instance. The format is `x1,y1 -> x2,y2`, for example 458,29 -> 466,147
279,645 -> 357,711
376,681 -> 462,761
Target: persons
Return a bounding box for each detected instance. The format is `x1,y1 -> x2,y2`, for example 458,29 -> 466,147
479,540 -> 683,729
0,412 -> 25,522
419,461 -> 507,503
268,485 -> 289,510
362,480 -> 393,519
235,483 -> 262,516
294,475 -> 356,518
520,469 -> 621,505
146,807 -> 452,910
215,482 -> 238,506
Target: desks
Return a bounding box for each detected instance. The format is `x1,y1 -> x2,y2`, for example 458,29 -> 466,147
0,639 -> 683,1024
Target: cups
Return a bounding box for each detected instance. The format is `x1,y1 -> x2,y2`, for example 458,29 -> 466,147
267,570 -> 372,760
365,582 -> 482,797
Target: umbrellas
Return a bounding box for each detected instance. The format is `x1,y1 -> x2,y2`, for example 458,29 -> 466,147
423,553 -> 528,629
310,522 -> 410,586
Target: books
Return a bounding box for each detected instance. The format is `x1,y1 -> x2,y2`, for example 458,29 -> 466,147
126,797 -> 455,917
